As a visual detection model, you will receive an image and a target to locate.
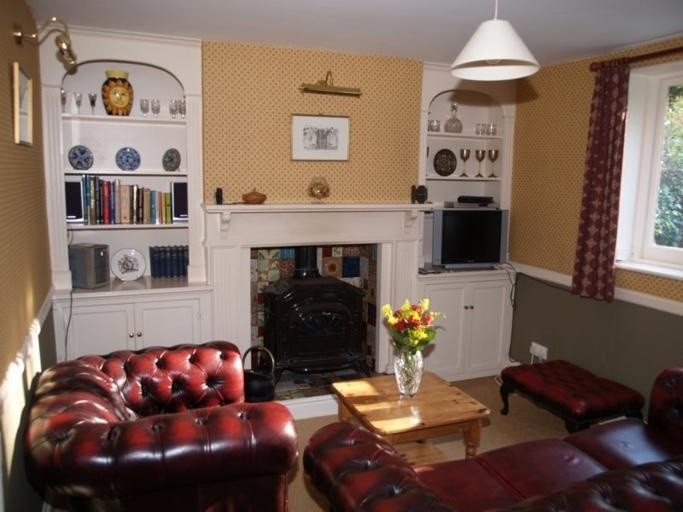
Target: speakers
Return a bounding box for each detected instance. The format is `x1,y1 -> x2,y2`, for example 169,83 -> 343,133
64,181 -> 84,224
171,181 -> 189,223
68,243 -> 111,289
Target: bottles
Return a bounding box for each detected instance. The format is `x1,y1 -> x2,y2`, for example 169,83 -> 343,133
444,104 -> 462,133
216,188 -> 222,204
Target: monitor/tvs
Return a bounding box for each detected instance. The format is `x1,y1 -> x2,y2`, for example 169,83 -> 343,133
432,209 -> 509,271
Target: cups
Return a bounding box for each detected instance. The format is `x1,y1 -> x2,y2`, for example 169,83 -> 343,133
431,120 -> 440,131
475,123 -> 499,135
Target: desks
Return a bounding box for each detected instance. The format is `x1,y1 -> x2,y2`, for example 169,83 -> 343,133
330,370 -> 491,461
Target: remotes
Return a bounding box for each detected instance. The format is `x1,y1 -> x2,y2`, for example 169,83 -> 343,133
419,268 -> 426,274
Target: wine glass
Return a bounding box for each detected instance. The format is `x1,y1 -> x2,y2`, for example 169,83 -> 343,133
60,90 -> 96,114
139,98 -> 185,119
459,148 -> 499,177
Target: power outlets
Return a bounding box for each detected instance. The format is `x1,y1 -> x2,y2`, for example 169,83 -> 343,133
529,341 -> 547,360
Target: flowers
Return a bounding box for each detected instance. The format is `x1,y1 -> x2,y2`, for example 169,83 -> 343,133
380,298 -> 447,352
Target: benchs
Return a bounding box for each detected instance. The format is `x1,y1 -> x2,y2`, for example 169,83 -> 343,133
499,357 -> 645,433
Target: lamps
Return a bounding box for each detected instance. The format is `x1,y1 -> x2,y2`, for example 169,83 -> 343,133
448,0 -> 541,82
9,15 -> 77,71
297,70 -> 362,96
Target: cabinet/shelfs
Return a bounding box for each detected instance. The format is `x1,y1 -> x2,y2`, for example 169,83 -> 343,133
414,270 -> 517,383
424,130 -> 503,182
60,110 -> 192,232
50,285 -> 213,362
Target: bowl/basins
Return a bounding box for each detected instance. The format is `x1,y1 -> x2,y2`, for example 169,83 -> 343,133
242,192 -> 266,204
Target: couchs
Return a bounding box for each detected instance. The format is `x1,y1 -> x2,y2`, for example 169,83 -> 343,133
301,368 -> 681,510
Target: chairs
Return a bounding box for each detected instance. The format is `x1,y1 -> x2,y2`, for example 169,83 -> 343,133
23,337 -> 303,511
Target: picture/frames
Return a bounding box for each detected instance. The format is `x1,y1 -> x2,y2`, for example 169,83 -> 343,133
11,62 -> 34,148
289,113 -> 352,162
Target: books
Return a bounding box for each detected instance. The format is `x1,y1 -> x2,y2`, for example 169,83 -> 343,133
79,174 -> 171,226
149,245 -> 187,279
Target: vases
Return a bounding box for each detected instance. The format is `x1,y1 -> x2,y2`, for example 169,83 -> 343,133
391,349 -> 424,396
100,70 -> 134,116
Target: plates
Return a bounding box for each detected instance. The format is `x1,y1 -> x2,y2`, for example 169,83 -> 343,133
162,149 -> 180,172
111,249 -> 146,281
67,146 -> 93,169
115,146 -> 140,171
434,148 -> 456,176
